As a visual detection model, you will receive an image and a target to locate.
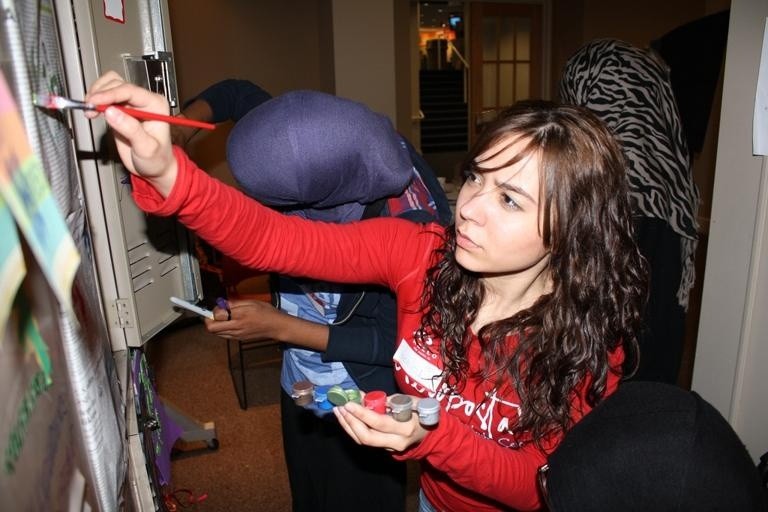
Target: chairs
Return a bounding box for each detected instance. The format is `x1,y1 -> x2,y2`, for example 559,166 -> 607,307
221,255 -> 287,410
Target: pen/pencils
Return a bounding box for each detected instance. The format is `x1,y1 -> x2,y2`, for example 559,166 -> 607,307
168,294 -> 219,321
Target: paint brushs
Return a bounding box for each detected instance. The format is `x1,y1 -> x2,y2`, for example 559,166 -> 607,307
30,90 -> 215,131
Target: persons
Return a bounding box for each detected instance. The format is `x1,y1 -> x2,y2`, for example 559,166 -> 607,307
82,41 -> 768,512
